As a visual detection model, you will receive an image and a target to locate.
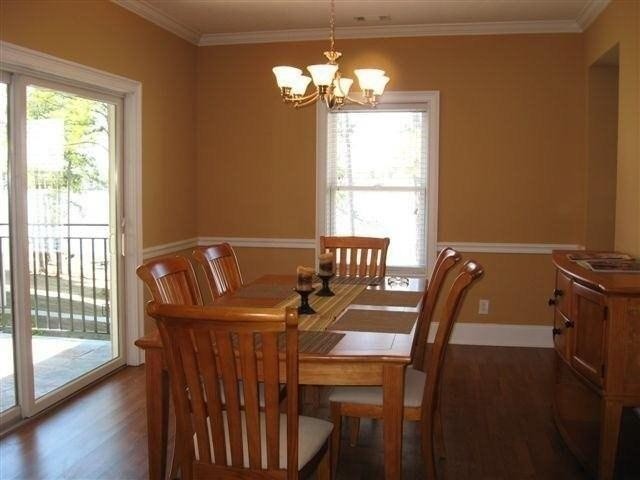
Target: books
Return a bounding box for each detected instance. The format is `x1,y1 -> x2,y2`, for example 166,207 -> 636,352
565,250 -> 640,275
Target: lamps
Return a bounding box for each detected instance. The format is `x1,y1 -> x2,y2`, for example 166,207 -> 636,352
272,0 -> 390,111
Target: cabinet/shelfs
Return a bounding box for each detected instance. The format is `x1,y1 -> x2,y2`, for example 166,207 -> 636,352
548,249 -> 640,480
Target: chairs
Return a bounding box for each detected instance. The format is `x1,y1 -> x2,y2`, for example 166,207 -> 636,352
326,258 -> 485,480
190,242 -> 245,301
320,237 -> 391,279
408,246 -> 460,368
135,254 -> 285,480
143,299 -> 335,480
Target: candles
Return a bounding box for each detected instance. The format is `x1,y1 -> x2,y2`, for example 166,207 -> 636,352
297,265 -> 314,292
319,248 -> 336,279
